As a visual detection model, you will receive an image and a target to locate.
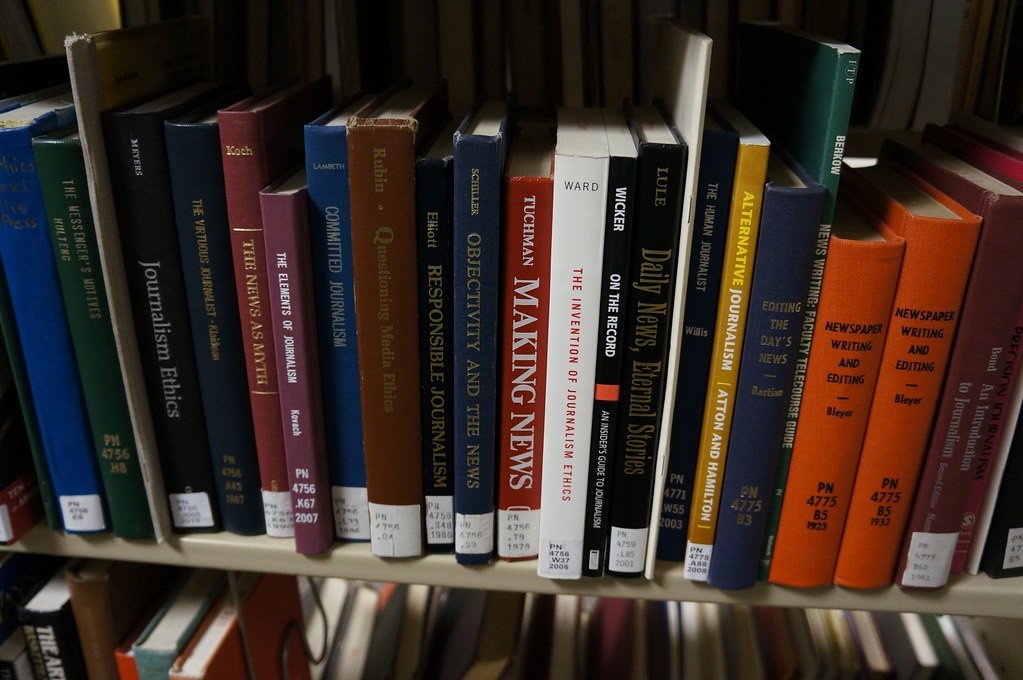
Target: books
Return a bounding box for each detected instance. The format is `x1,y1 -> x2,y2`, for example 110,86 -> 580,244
1,1 -> 1023,680
